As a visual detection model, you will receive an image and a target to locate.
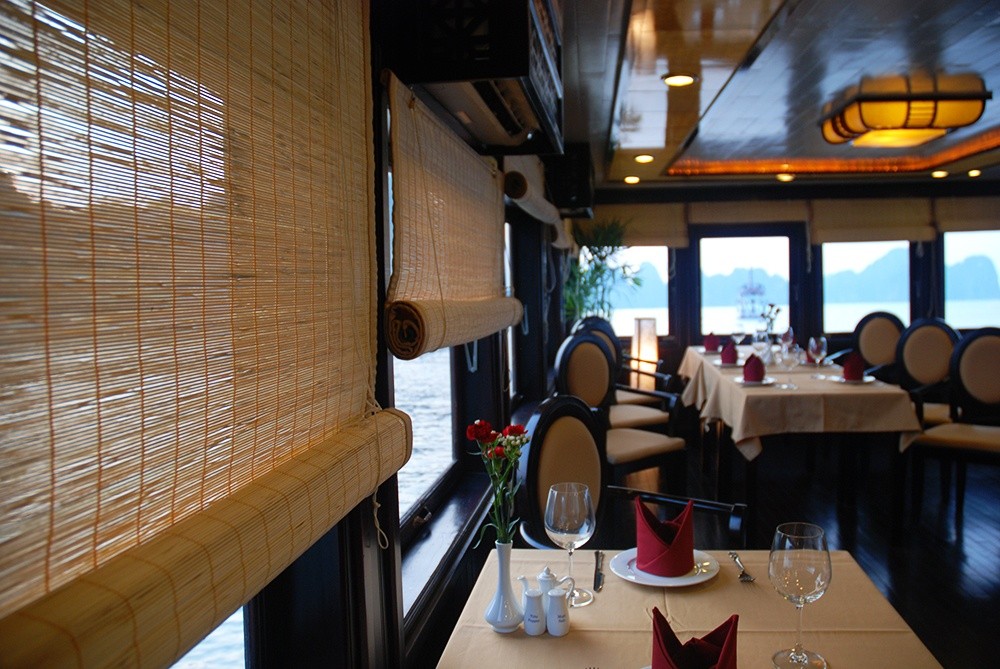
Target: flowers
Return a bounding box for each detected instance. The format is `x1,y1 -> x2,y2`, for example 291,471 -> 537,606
466,419 -> 530,550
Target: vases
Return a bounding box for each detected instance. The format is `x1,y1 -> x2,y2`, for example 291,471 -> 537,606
485,540 -> 524,633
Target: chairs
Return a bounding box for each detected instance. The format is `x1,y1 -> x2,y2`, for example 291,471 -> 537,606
828,311 -> 1000,542
514,316 -> 747,551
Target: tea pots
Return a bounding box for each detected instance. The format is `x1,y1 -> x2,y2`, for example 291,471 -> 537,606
516,567 -> 575,615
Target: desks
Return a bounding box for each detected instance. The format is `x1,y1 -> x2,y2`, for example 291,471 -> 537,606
436,548 -> 944,669
678,344 -> 922,546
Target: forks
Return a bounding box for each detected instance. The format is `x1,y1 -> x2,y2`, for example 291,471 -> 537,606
728,551 -> 755,583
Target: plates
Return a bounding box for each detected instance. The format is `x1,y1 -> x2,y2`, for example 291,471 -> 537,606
609,547 -> 720,588
698,346 -> 875,385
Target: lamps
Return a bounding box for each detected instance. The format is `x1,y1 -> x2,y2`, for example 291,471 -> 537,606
819,91 -> 993,148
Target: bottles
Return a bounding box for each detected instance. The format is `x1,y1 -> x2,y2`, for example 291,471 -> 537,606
524,589 -> 546,636
546,589 -> 570,637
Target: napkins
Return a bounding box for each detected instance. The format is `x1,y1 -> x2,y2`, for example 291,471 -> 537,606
651,606 -> 739,669
703,333 -> 865,382
633,495 -> 694,577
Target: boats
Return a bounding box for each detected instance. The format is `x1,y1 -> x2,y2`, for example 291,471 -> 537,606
738,282 -> 768,320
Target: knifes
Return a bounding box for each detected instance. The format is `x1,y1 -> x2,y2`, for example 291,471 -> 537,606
593,550 -> 605,591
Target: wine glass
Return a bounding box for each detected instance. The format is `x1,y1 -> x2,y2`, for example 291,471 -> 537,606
752,330 -> 770,377
775,326 -> 794,360
731,324 -> 747,358
776,343 -> 800,391
807,337 -> 827,381
767,522 -> 833,669
544,482 -> 596,608
